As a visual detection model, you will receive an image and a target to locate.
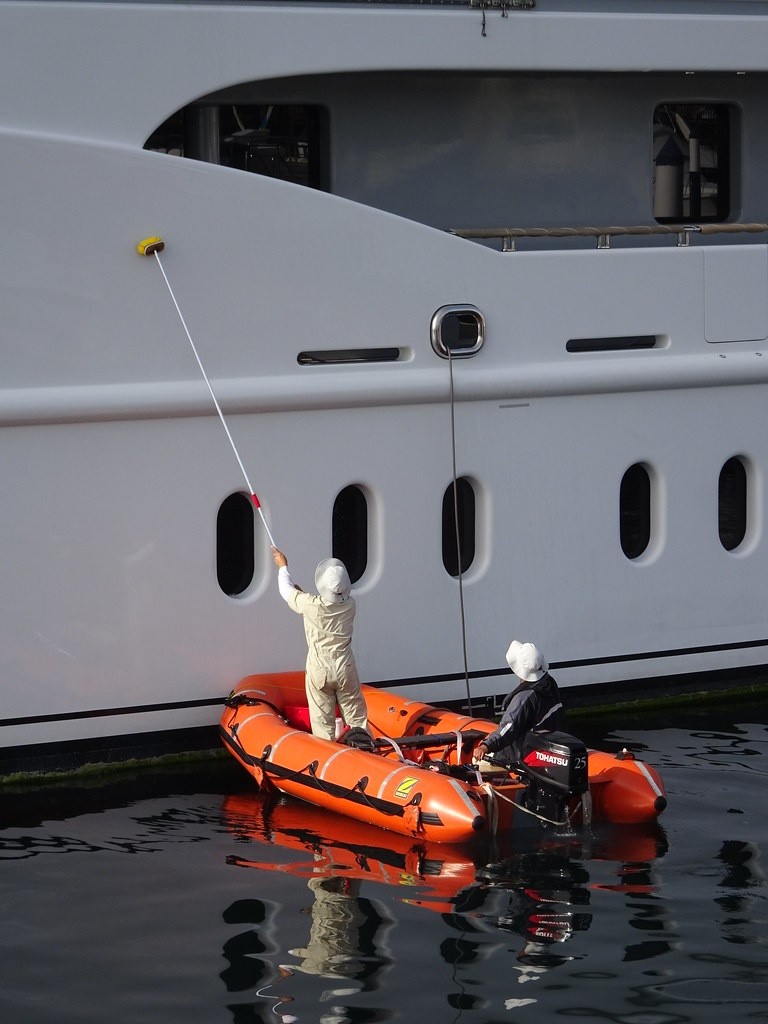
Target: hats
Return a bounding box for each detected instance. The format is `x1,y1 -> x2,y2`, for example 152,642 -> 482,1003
315,558 -> 351,604
506,640 -> 549,682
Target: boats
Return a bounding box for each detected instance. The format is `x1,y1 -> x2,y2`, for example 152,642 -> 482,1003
211,671 -> 669,849
0,1 -> 768,741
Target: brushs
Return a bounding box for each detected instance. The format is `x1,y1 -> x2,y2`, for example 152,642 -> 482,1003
136,236 -> 297,590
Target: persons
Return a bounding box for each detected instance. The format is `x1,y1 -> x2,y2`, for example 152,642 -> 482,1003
473,639 -> 565,772
271,544 -> 375,741
